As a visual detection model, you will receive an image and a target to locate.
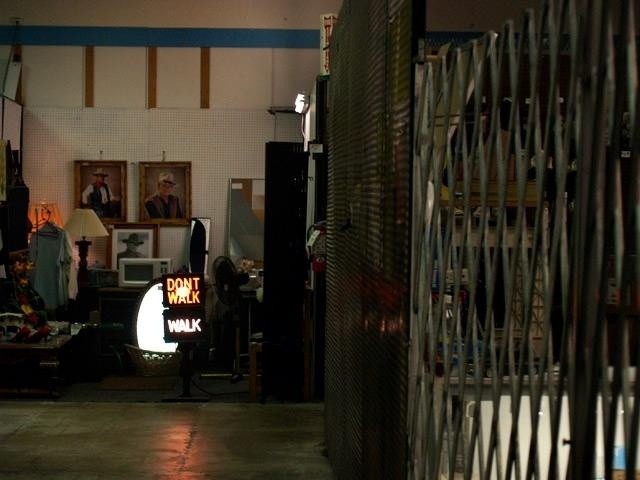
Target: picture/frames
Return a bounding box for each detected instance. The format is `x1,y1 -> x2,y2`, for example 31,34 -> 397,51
72,161 -> 192,270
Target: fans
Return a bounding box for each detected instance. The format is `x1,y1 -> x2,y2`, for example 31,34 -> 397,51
199,256 -> 259,384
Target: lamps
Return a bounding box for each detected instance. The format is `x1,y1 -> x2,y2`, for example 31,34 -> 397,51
59,205 -> 105,317
294,89 -> 309,116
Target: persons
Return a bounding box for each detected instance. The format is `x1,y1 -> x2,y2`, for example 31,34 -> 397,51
117,233 -> 146,270
143,171 -> 184,218
30,209 -> 57,232
81,167 -> 121,217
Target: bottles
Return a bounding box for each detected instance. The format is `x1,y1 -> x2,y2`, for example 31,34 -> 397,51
426,293 -> 455,376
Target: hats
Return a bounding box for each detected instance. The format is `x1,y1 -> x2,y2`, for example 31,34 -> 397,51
92,168 -> 109,177
122,233 -> 144,245
158,171 -> 176,186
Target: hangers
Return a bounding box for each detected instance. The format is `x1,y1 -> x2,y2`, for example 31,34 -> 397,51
29,208 -> 62,240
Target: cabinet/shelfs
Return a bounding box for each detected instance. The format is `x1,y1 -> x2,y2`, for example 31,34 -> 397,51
1,334 -> 73,401
425,190 -> 635,480
265,142 -> 313,399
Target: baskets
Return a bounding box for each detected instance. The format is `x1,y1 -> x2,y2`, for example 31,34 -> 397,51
125,343 -> 181,377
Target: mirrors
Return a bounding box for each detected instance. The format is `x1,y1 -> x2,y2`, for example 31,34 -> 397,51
128,277 -> 180,362
227,176 -> 265,272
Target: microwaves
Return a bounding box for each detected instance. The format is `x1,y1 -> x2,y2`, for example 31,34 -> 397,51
117,257 -> 172,287
